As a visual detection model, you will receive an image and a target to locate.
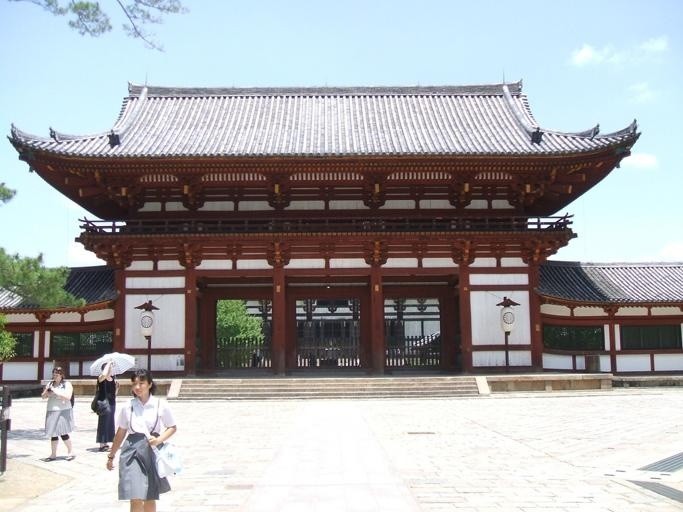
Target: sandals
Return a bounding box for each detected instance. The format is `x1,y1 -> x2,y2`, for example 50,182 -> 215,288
98,446 -> 111,451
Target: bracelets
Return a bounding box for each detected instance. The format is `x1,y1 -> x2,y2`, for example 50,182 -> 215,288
107,453 -> 116,459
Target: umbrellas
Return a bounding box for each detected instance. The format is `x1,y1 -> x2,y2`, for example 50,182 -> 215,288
89,352 -> 135,381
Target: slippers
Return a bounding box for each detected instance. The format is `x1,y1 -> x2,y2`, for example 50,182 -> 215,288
44,458 -> 56,461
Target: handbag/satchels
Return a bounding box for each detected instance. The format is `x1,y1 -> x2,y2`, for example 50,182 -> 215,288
91,394 -> 111,416
154,442 -> 183,479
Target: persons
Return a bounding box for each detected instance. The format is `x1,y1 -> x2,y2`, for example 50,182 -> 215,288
94,363 -> 119,450
105,369 -> 176,512
39,367 -> 79,461
